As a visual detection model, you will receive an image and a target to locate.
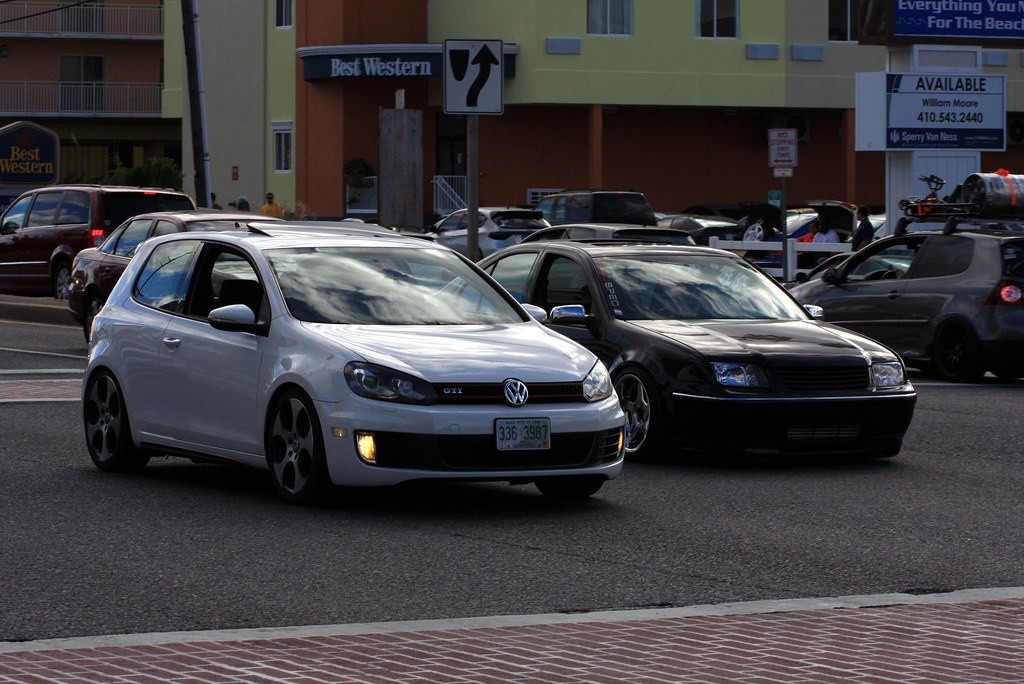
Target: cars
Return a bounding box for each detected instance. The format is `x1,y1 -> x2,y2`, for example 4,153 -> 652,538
0,183 -> 200,302
64,210 -> 302,354
78,223 -> 629,508
282,179 -> 927,298
422,243 -> 918,461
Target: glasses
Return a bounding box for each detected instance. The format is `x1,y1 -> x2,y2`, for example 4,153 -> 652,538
267,197 -> 273,199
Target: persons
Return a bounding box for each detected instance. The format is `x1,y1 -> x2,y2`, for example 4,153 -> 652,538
851,205 -> 875,252
260,192 -> 284,218
211,192 -> 223,211
235,196 -> 251,212
795,216 -> 840,243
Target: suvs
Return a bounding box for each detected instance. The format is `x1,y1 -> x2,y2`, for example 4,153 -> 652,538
781,215 -> 1024,381
